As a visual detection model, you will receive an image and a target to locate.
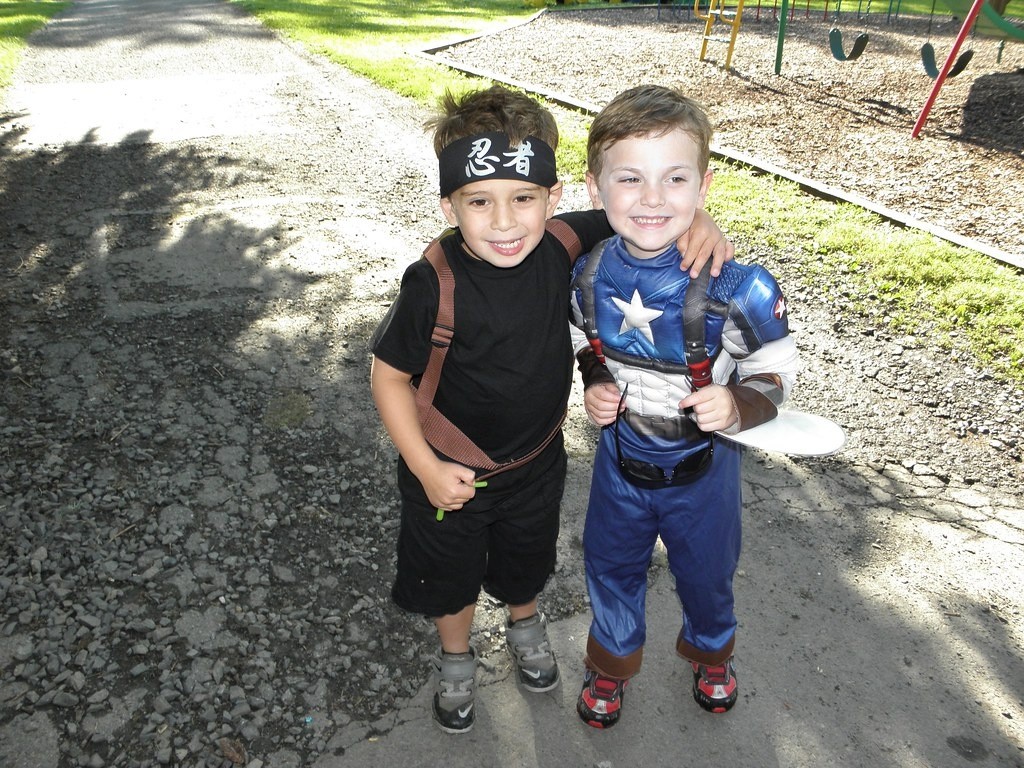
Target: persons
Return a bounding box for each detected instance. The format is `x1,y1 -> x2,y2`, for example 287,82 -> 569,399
569,85 -> 797,728
369,88 -> 733,732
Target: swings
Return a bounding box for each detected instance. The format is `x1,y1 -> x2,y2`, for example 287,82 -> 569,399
827,0 -> 872,62
919,0 -> 981,80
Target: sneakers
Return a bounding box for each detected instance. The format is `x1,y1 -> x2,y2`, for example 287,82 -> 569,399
688,654 -> 740,714
505,613 -> 561,693
576,659 -> 627,729
430,641 -> 479,735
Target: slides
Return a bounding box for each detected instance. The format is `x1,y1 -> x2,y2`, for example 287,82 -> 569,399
945,0 -> 1024,44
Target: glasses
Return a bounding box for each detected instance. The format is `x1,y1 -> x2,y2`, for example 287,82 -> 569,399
610,384 -> 717,488
